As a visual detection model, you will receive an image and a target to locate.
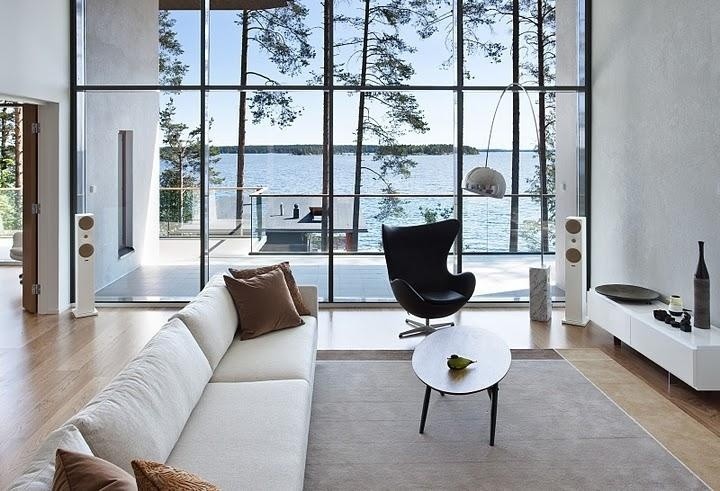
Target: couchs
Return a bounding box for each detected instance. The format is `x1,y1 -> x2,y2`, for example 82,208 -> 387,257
5,424 -> 97,491
12,271 -> 320,491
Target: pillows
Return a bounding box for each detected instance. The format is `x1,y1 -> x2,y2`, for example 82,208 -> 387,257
52,447 -> 138,491
62,316 -> 214,483
228,261 -> 311,316
223,268 -> 304,341
168,271 -> 239,374
130,456 -> 222,491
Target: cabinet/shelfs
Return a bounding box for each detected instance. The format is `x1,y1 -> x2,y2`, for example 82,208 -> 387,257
586,286 -> 720,392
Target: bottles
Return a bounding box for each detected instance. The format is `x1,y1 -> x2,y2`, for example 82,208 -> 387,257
694,240 -> 712,329
668,294 -> 684,316
293,203 -> 300,218
279,202 -> 285,216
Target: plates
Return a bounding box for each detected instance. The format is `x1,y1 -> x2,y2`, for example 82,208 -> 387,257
594,284 -> 662,302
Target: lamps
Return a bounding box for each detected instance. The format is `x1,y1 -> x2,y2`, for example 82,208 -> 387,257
460,81 -> 552,322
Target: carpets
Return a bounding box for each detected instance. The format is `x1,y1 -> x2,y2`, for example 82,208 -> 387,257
301,347 -> 720,491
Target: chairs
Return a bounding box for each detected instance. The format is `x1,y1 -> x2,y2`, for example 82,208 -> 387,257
9,231 -> 23,284
381,218 -> 476,339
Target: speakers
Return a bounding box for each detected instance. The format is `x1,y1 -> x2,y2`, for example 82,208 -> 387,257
70,214 -> 98,318
562,216 -> 590,327
